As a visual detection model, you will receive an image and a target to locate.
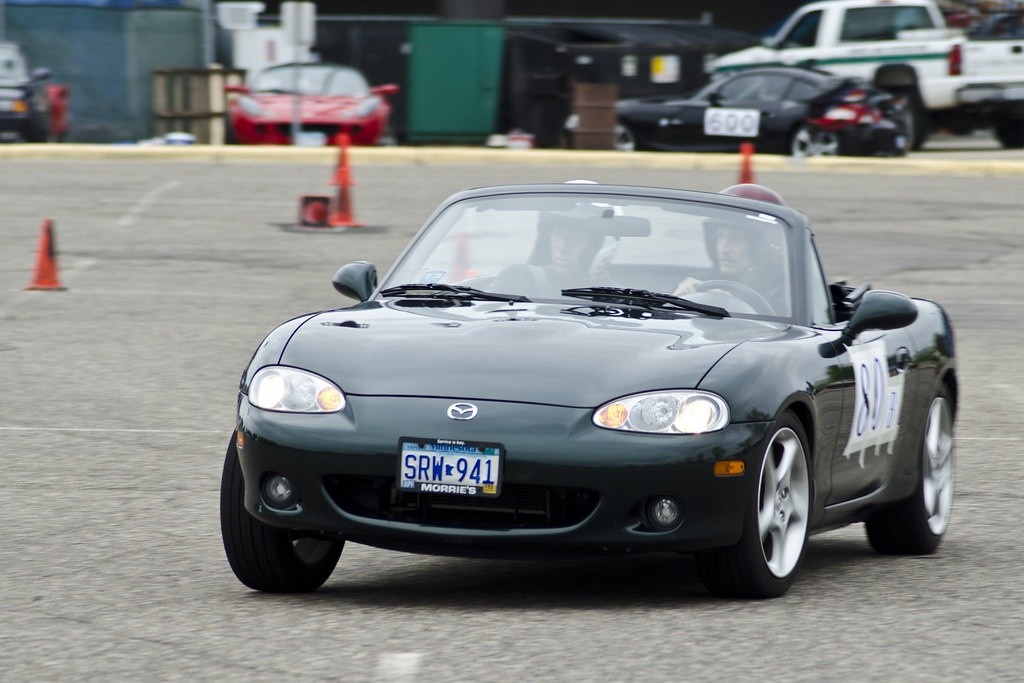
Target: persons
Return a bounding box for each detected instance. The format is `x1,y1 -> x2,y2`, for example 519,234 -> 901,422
489,179 -> 623,298
672,184 -> 791,317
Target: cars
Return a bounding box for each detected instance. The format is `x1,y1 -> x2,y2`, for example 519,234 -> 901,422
313,9 -> 757,144
940,2 -> 978,28
969,11 -> 1024,42
612,65 -> 911,164
1,41 -> 50,143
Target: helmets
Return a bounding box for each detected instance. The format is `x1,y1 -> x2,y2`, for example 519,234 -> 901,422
529,179 -> 623,276
704,183 -> 787,272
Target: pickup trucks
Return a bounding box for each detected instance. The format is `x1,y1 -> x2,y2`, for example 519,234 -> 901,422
700,0 -> 1023,147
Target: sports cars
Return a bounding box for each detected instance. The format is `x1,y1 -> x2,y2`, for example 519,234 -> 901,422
218,174 -> 962,606
221,59 -> 401,146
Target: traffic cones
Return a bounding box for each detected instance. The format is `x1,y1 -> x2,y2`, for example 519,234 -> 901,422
733,139 -> 754,185
25,217 -> 72,289
324,147 -> 361,227
